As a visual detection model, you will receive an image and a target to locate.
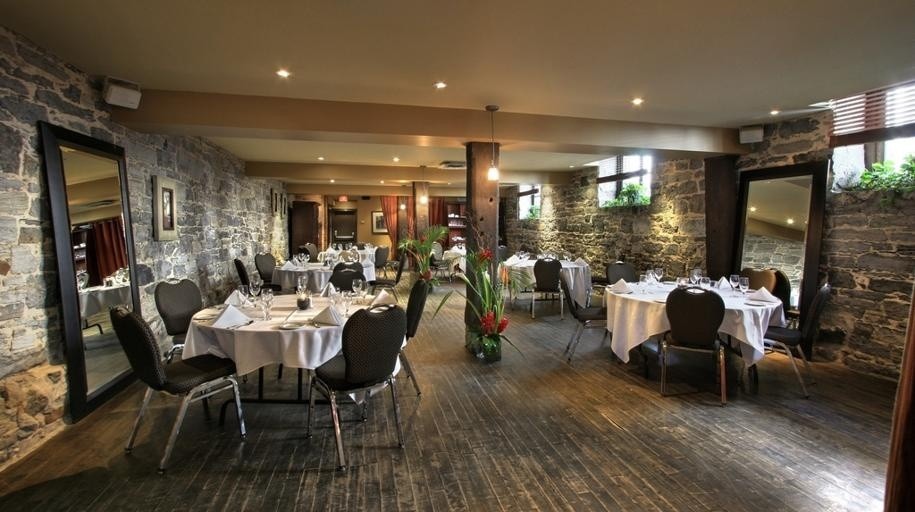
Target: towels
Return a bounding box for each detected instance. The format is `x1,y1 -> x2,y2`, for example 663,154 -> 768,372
749,286 -> 777,303
225,290 -> 253,308
370,289 -> 398,306
280,260 -> 296,270
608,278 -> 633,292
320,282 -> 337,298
574,256 -> 588,267
715,276 -> 732,290
312,305 -> 344,326
210,304 -> 252,328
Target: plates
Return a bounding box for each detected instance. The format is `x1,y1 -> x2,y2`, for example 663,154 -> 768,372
193,314 -> 219,320
653,298 -> 666,303
745,301 -> 766,307
280,322 -> 306,329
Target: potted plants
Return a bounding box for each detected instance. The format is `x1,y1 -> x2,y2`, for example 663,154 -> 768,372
619,184 -> 643,206
398,224 -> 449,289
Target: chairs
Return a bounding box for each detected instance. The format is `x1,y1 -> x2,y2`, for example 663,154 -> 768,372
603,261 -> 640,340
298,242 -> 320,263
370,243 -> 390,278
366,253 -> 407,304
333,261 -> 368,273
107,304 -> 249,476
558,268 -> 615,363
498,244 -> 518,312
746,281 -> 834,400
154,278 -> 249,401
306,302 -> 409,473
233,258 -> 283,296
334,242 -> 350,251
529,257 -> 564,321
659,285 -> 729,406
768,267 -> 801,330
395,278 -> 431,396
738,266 -> 776,293
351,242 -> 365,250
254,251 -> 278,284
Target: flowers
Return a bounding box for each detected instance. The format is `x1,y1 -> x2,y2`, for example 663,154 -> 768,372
429,245 -> 533,338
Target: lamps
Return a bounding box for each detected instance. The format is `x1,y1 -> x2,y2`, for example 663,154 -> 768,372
485,105 -> 500,181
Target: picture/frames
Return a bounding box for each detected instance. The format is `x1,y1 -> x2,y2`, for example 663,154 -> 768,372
281,192 -> 287,219
152,175 -> 177,241
271,188 -> 280,217
371,211 -> 389,235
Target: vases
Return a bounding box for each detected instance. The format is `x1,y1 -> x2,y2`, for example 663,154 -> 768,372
472,338 -> 502,362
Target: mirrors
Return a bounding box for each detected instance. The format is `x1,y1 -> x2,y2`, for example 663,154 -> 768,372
734,161 -> 829,360
35,120 -> 142,425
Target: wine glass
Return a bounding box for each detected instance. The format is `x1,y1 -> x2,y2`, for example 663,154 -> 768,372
296,275 -> 308,295
676,277 -> 689,290
537,253 -> 558,262
639,274 -> 647,295
345,243 -> 349,251
349,242 -> 353,250
292,253 -> 310,268
516,250 -> 531,260
458,243 -> 461,249
77,278 -> 84,292
261,287 -> 274,321
739,277 -> 750,298
328,295 -> 337,307
116,274 -> 122,284
337,243 -> 343,252
567,252 -> 573,262
654,267 -> 664,284
237,284 -> 249,310
696,266 -> 702,286
248,280 -> 264,309
352,279 -> 363,298
646,268 -> 655,284
562,249 -> 568,261
341,290 -> 352,318
700,277 -> 710,290
729,274 -> 740,296
123,269 -> 129,283
689,269 -> 696,287
332,243 -> 336,250
119,268 -> 123,284
